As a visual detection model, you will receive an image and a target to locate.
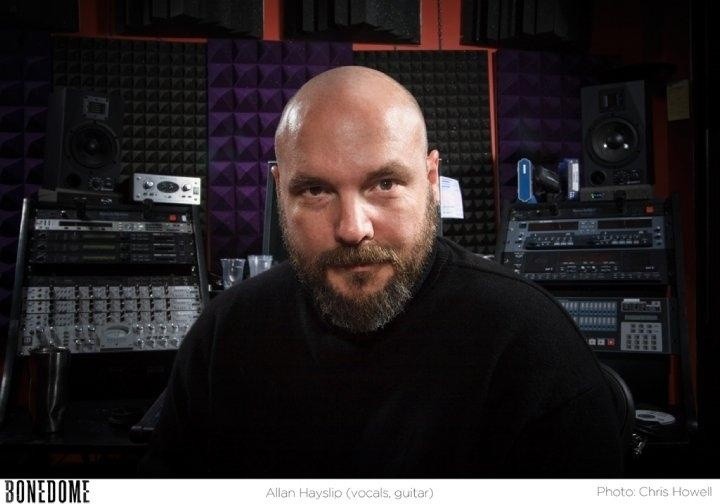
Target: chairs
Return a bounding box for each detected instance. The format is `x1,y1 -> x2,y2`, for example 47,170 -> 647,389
604,363 -> 637,449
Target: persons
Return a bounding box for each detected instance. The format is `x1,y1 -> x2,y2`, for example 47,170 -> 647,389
123,63 -> 631,478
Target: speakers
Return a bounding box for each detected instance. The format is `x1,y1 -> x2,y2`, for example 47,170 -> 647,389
579,78 -> 652,186
44,87 -> 123,194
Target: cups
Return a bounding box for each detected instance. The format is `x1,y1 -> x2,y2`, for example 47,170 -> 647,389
247,253 -> 274,277
217,256 -> 245,292
